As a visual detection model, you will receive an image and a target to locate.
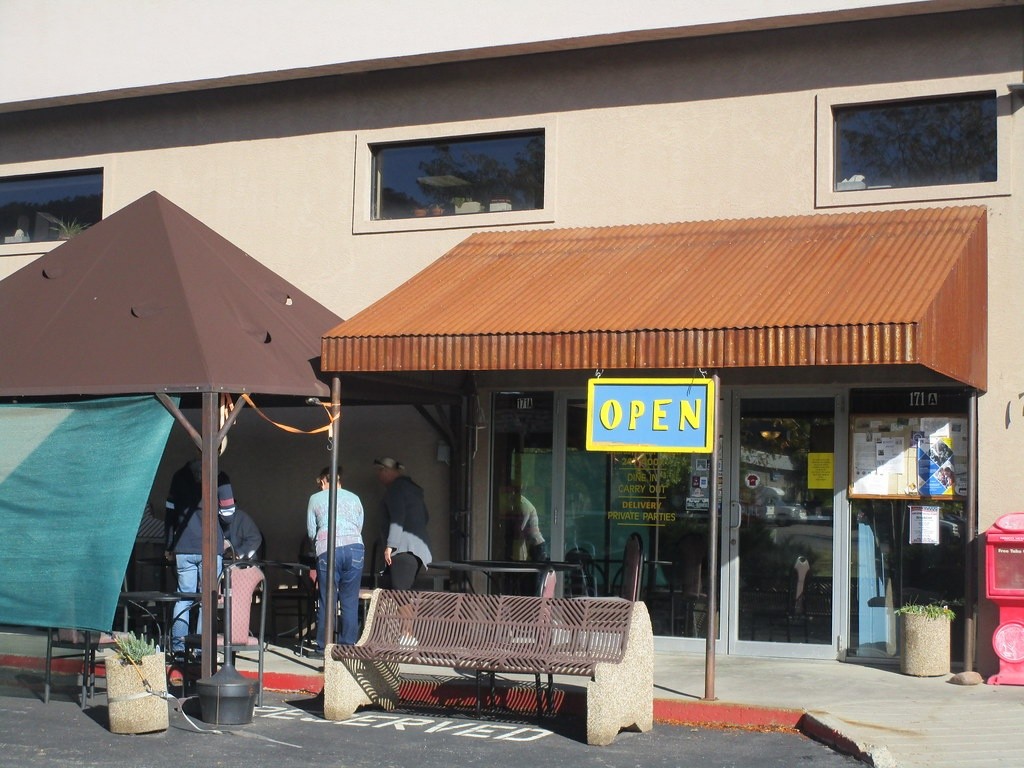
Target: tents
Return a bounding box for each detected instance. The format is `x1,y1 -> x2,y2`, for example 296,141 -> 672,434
1,190 -> 358,685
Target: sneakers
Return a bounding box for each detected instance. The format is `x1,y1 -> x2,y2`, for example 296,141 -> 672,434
307,650 -> 324,660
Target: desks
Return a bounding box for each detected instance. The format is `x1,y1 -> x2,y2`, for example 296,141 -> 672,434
119,591 -> 202,695
584,559 -> 673,596
427,560 -> 582,594
136,558 -> 176,635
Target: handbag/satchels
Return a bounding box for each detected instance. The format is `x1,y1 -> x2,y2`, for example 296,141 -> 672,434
224,539 -> 236,562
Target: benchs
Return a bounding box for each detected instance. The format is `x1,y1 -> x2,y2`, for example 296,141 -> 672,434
324,588 -> 654,746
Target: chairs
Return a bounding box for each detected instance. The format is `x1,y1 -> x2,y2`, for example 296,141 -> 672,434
752,556 -> 811,643
475,569 -> 557,725
42,627 -> 119,710
181,561 -> 267,707
566,547 -> 598,597
655,532 -> 708,636
618,531 -> 645,600
271,534 -> 317,645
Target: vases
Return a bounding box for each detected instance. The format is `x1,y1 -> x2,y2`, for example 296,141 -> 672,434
490,204 -> 512,211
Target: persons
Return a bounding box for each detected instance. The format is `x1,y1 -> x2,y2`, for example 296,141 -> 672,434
305,465 -> 366,660
164,431 -> 236,684
373,455 -> 432,649
507,478 -> 548,597
216,501 -> 263,573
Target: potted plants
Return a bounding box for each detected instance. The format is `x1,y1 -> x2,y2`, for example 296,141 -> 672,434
413,199 -> 447,217
450,197 -> 486,214
104,631 -> 169,733
894,595 -> 957,677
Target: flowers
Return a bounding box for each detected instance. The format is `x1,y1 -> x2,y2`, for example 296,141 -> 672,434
491,199 -> 512,204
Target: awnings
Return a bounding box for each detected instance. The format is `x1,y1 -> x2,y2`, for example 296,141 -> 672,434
318,205 -> 990,398
